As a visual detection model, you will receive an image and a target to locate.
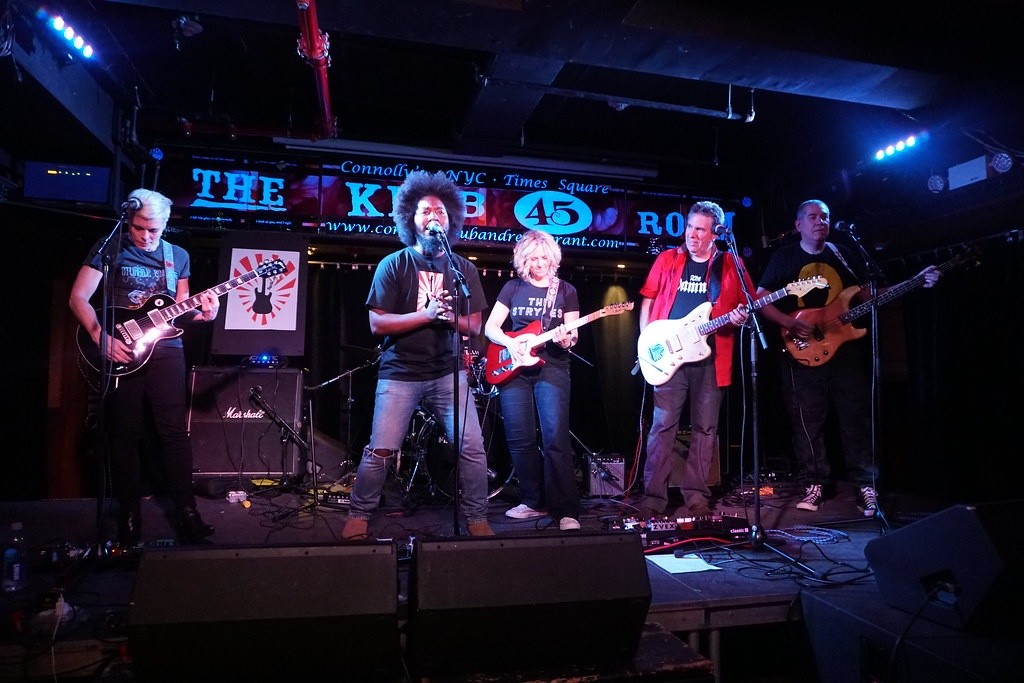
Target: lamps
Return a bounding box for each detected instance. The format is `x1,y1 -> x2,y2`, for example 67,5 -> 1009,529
602,274 -> 629,314
927,151 -> 1011,196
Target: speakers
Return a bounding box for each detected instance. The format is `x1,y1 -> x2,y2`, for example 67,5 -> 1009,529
582,452 -> 626,498
403,528 -> 652,676
123,540 -> 400,683
864,499 -> 1024,631
305,426 -> 361,484
186,364 -> 304,478
666,431 -> 721,488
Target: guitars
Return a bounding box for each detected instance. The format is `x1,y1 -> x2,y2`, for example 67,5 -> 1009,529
780,241 -> 981,369
637,274 -> 831,388
75,258 -> 288,377
485,298 -> 636,386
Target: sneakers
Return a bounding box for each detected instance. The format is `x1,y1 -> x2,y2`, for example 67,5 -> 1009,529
796,485 -> 825,511
858,487 -> 877,516
505,504 -> 548,520
560,516 -> 580,529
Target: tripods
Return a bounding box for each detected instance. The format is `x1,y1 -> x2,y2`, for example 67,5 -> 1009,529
674,233 -> 827,582
808,229 -> 932,535
273,392 -> 351,523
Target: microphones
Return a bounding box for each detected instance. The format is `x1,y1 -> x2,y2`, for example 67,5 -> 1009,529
122,198 -> 140,209
487,469 -> 498,483
715,225 -> 731,234
428,221 -> 446,234
250,385 -> 262,392
835,221 -> 856,230
599,470 -> 619,481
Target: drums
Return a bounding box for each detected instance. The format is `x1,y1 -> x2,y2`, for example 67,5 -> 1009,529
478,356 -> 500,400
418,405 -> 515,502
462,350 -> 480,394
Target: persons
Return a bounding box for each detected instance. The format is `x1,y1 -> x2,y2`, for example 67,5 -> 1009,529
756,199 -> 943,517
639,201 -> 758,517
342,169 -> 495,539
483,230 -> 581,530
68,187 -> 220,540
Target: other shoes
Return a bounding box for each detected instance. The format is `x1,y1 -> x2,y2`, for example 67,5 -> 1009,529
688,504 -> 713,515
176,507 -> 216,543
468,521 -> 496,535
342,514 -> 367,541
638,507 -> 659,521
116,505 -> 142,546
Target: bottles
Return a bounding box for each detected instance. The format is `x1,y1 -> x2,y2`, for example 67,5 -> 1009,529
2,522 -> 28,591
